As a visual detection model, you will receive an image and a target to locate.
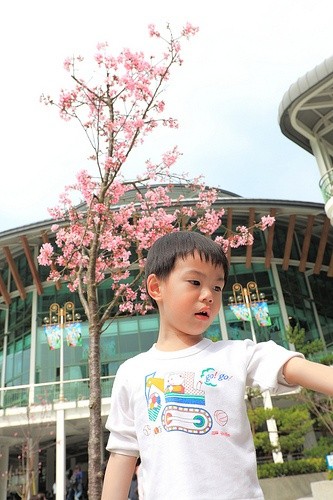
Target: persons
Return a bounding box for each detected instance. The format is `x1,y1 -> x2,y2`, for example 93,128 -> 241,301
66,465 -> 84,500
101,231 -> 333,500
